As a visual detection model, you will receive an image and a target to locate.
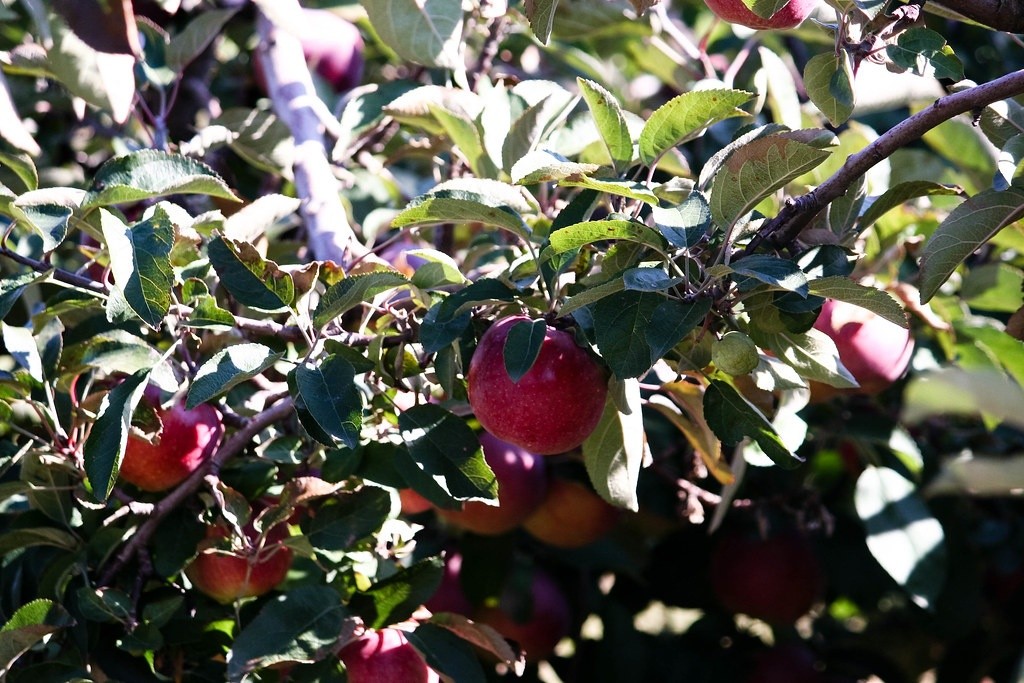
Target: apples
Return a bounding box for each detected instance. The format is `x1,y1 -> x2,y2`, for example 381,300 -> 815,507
82,0 -> 916,683
